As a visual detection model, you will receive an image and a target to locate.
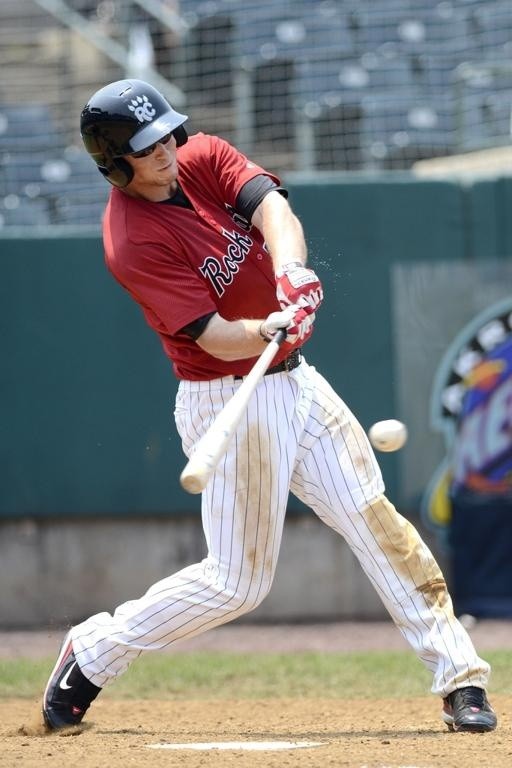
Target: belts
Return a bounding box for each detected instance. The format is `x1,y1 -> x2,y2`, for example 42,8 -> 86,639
235,347 -> 303,382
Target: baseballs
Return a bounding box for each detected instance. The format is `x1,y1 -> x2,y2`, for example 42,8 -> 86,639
369,419 -> 407,451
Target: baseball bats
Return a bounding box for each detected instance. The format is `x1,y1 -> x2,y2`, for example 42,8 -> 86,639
179,329 -> 288,495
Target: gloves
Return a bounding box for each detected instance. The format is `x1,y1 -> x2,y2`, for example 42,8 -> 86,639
260,262 -> 324,354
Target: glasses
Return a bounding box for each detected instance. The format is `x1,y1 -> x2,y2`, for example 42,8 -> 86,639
130,133 -> 172,159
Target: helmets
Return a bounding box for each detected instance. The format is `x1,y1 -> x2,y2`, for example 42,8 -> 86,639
80,78 -> 190,189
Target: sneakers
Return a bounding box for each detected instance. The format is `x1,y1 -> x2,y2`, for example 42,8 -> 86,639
439,685 -> 498,733
42,628 -> 102,733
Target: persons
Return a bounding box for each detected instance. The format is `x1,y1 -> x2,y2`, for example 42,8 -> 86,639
38,79 -> 498,734
